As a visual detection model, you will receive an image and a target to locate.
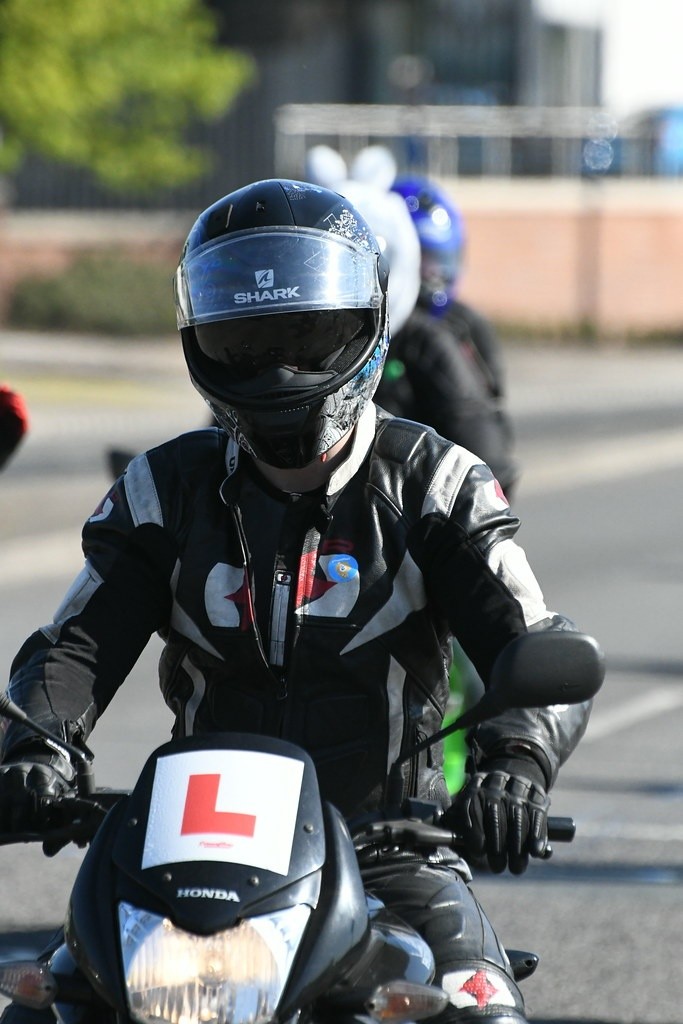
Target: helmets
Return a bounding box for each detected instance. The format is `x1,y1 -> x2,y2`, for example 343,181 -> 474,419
173,178 -> 389,470
308,143 -> 422,337
391,173 -> 463,317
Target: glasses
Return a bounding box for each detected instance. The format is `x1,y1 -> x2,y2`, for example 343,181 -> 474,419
195,309 -> 367,385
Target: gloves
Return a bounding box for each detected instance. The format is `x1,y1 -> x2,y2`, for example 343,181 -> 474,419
0,748 -> 81,857
433,753 -> 551,875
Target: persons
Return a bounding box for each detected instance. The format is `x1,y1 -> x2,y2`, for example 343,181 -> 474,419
0,178 -> 594,1024
311,176 -> 518,505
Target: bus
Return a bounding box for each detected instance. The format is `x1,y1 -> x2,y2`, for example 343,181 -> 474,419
275,97 -> 681,205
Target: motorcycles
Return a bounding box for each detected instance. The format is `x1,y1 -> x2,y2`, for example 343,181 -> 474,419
0,630 -> 610,1024
